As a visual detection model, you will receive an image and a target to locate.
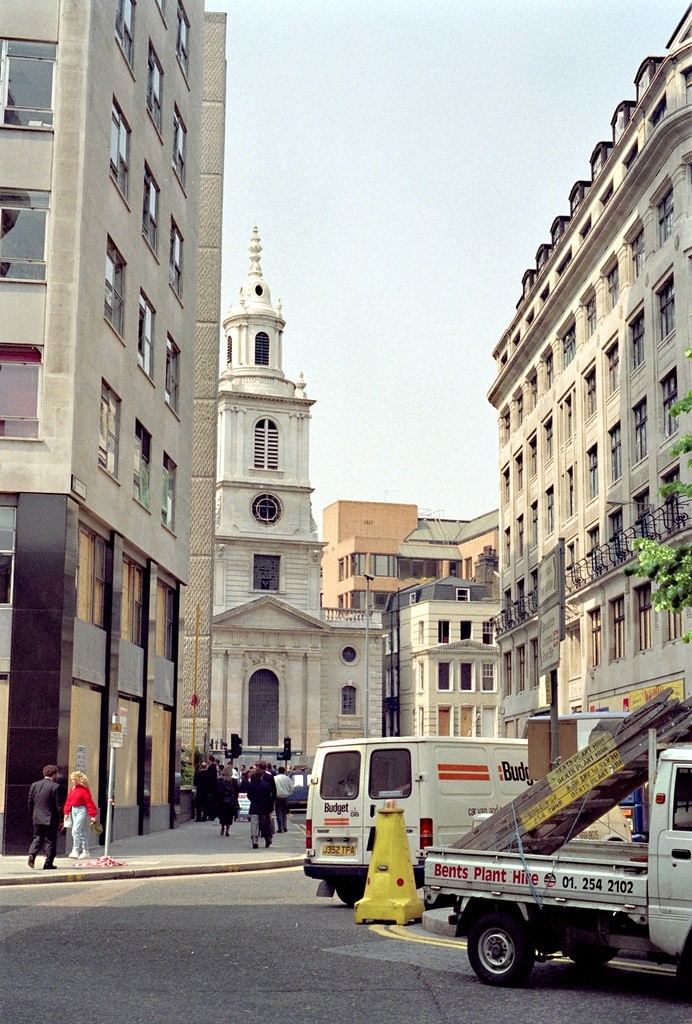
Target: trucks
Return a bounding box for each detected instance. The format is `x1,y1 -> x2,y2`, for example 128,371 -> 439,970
522,711 -> 692,843
422,747 -> 692,1002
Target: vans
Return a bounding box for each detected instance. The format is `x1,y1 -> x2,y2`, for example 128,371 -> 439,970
303,738 -> 633,906
286,768 -> 312,812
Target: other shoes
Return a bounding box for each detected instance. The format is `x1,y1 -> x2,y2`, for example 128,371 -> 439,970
277,830 -> 282,833
78,852 -> 91,859
68,852 -> 79,858
253,843 -> 258,849
28,854 -> 35,868
43,863 -> 57,870
284,828 -> 287,832
220,828 -> 224,835
265,840 -> 271,847
226,833 -> 229,837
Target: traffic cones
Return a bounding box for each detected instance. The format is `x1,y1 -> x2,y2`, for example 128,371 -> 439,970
352,799 -> 427,926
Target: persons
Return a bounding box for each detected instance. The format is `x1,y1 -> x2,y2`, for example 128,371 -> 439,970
28,765 -> 64,870
63,771 -> 98,859
193,756 -> 294,849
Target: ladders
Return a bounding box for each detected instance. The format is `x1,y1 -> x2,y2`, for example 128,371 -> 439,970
422,686 -> 692,903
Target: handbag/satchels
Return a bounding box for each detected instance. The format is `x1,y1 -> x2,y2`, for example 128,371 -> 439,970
64,812 -> 73,829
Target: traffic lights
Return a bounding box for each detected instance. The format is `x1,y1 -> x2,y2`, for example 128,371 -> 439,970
224,733 -> 242,758
276,738 -> 292,760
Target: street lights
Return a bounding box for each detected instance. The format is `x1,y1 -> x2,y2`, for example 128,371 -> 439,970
361,572 -> 375,737
